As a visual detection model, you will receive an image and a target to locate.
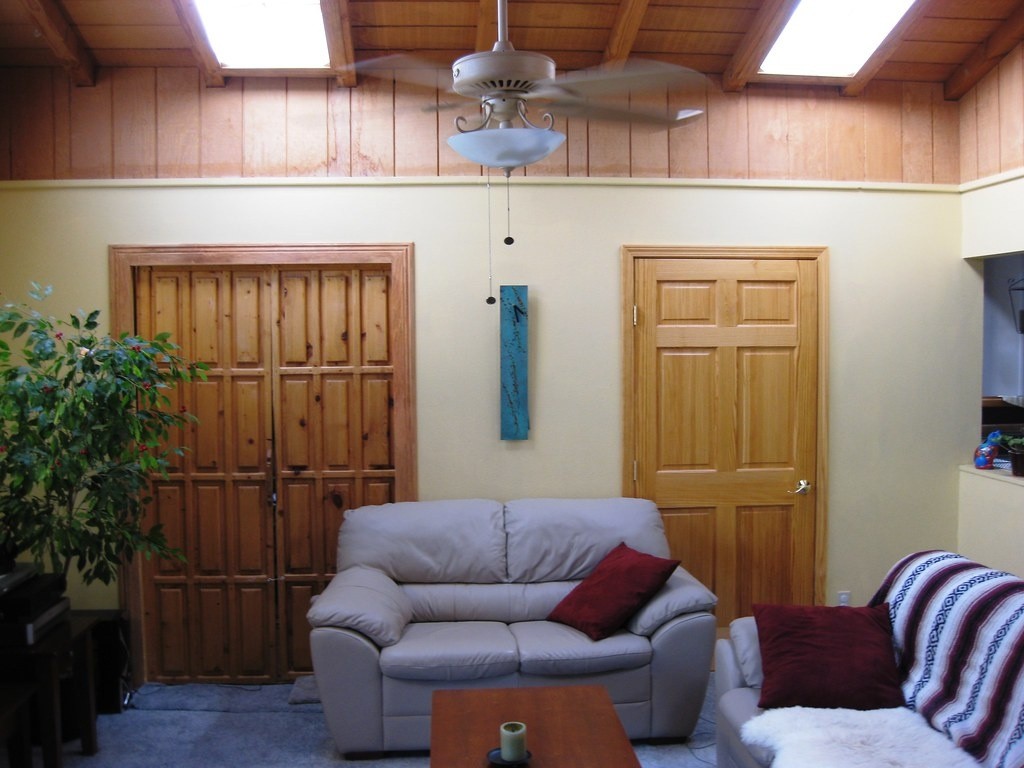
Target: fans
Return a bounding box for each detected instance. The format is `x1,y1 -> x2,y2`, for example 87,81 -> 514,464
335,0 -> 726,305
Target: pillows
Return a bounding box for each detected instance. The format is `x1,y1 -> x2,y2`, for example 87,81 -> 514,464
750,602 -> 907,708
546,541 -> 682,643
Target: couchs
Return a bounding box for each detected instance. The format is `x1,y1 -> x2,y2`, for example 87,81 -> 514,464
308,497 -> 720,758
714,549 -> 1024,768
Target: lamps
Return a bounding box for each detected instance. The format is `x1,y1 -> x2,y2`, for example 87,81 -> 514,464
447,120 -> 566,304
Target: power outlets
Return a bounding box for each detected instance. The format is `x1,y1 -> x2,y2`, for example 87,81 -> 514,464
837,591 -> 851,606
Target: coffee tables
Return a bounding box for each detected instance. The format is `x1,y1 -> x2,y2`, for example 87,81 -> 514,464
430,684 -> 641,768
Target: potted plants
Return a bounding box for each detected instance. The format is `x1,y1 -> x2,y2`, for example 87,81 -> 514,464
991,435 -> 1024,477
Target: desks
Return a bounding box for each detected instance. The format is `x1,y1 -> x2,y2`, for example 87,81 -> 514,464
0,615 -> 98,768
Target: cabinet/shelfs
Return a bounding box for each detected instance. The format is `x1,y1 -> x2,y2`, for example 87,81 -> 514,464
957,464 -> 1024,578
